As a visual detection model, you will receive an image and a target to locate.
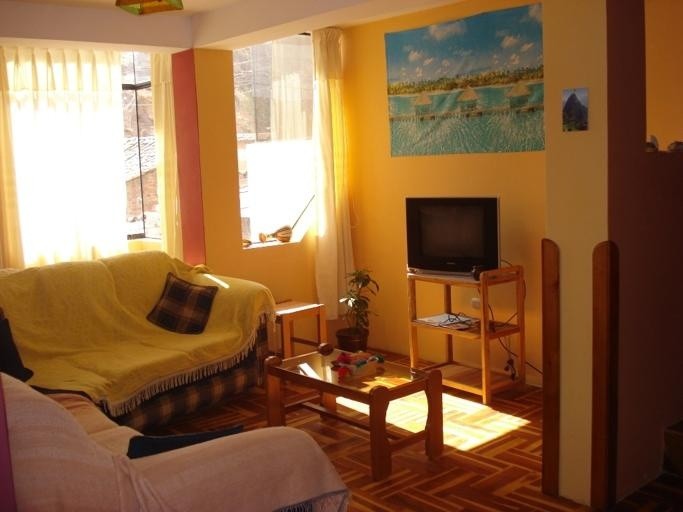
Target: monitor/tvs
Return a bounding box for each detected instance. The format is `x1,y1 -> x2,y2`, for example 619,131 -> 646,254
405,194 -> 501,277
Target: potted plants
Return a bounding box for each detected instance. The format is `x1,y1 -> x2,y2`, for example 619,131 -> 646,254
335,269 -> 379,353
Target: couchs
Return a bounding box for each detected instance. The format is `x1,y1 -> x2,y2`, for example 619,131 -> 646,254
0,250 -> 274,420
0,371 -> 351,512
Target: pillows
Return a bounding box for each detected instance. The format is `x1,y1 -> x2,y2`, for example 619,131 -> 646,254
146,272 -> 218,334
127,424 -> 244,459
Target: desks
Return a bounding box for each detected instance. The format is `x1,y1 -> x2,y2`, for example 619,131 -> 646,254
275,297 -> 328,360
406,264 -> 525,405
